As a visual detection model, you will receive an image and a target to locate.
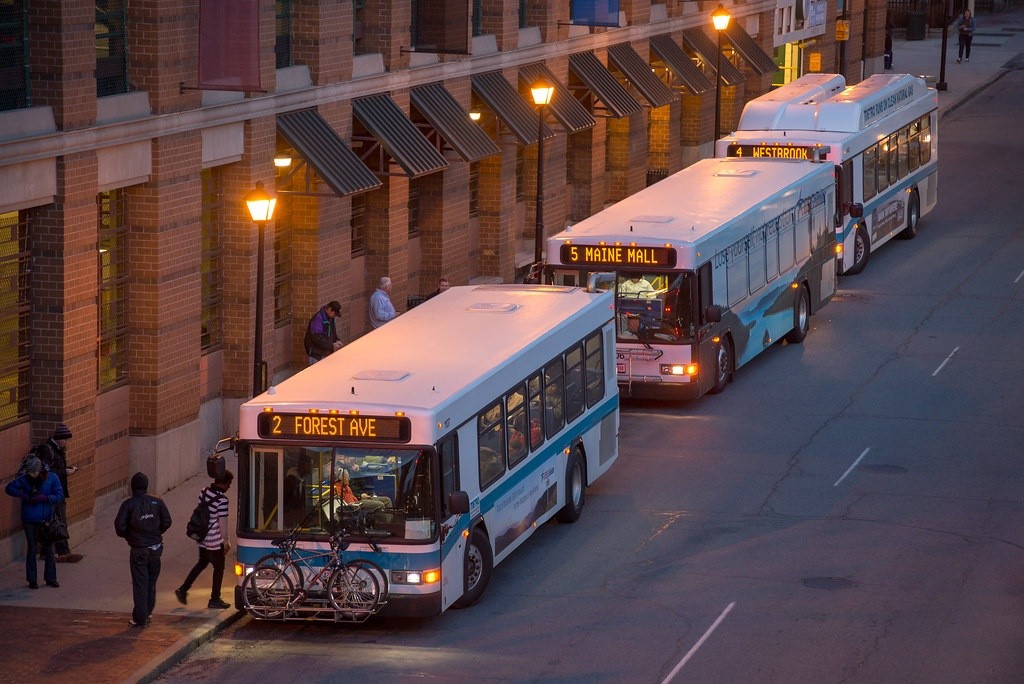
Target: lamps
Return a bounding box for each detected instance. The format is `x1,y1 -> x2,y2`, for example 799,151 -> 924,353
468,105 -> 481,120
273,151 -> 292,167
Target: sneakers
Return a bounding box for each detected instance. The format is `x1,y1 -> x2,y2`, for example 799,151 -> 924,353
175,588 -> 187,604
208,598 -> 230,608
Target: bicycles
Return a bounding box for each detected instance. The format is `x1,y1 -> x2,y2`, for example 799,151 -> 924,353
241,498 -> 388,620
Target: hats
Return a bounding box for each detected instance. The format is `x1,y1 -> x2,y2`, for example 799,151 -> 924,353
330,301 -> 342,317
25,453 -> 42,472
53,423 -> 72,439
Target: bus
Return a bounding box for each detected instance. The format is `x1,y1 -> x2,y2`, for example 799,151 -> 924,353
207,282 -> 618,615
715,73 -> 940,275
514,156 -> 838,401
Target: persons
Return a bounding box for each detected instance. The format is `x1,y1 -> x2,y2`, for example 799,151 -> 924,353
425,277 -> 450,301
37,425 -> 83,562
175,471 -> 234,610
369,277 -> 400,329
114,472 -> 171,628
5,456 -> 64,589
480,365 -> 583,484
955,9 -> 975,64
334,468 -> 394,521
886,9 -> 896,70
618,278 -> 656,298
284,456 -> 312,526
304,301 -> 343,365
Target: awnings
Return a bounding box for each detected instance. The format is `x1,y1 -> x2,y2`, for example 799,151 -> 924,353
520,17 -> 780,134
407,81 -> 502,163
470,69 -> 558,146
350,91 -> 450,179
276,106 -> 383,198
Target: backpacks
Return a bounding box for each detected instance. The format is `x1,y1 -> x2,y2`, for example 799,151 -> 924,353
186,487 -> 222,543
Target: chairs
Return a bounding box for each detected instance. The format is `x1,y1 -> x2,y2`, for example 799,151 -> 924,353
481,377 -> 586,454
363,463 -> 391,475
865,142 -> 922,188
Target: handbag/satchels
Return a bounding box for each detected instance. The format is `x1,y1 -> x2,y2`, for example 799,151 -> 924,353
47,496 -> 69,541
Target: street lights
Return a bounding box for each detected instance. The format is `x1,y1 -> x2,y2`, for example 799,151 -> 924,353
710,3 -> 737,158
528,75 -> 555,278
242,180 -> 277,398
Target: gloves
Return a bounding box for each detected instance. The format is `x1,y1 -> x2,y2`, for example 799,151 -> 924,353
30,495 -> 47,503
19,491 -> 30,501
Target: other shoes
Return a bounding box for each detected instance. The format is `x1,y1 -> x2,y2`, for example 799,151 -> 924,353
58,552 -> 82,562
129,617 -> 152,628
46,579 -> 59,588
29,581 -> 38,589
957,59 -> 961,63
966,59 -> 969,63
